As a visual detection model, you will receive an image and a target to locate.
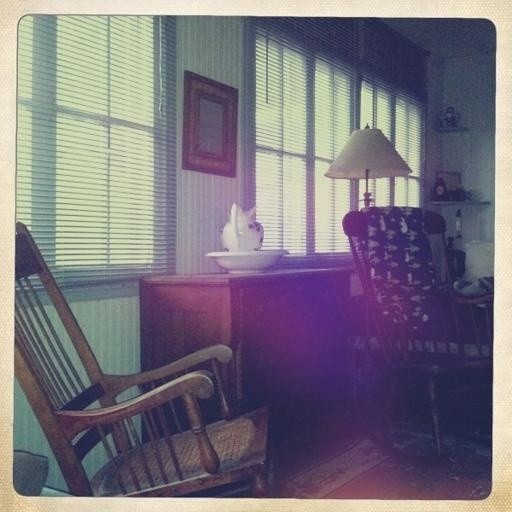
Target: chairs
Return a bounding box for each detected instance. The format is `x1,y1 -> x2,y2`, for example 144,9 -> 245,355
342,207 -> 493,454
17,221 -> 272,498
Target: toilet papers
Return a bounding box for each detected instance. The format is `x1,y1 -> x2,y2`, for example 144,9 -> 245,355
463,238 -> 493,282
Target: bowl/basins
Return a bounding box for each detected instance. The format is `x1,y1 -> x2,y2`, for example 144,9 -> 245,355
205,250 -> 290,274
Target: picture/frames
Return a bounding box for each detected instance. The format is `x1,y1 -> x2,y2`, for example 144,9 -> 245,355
181,70 -> 239,178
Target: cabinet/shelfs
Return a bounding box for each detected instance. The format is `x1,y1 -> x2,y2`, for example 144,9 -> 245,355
138,266 -> 354,456
429,124 -> 491,206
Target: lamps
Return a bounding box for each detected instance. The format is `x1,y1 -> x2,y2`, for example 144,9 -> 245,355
325,123 -> 413,207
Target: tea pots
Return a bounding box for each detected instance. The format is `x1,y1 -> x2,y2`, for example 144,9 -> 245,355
221,203 -> 262,252
